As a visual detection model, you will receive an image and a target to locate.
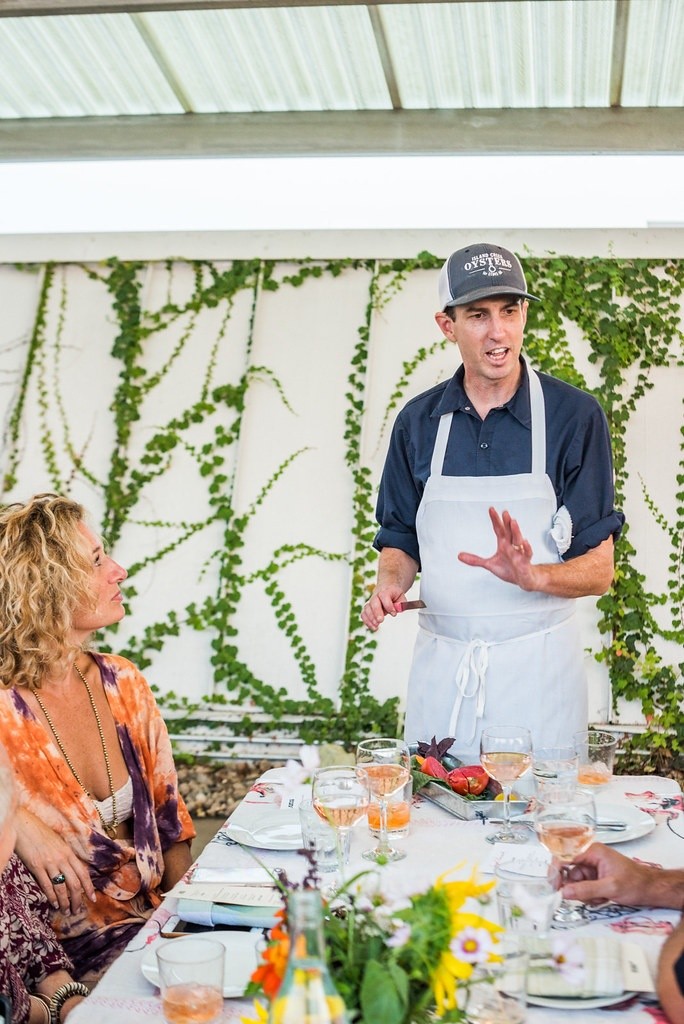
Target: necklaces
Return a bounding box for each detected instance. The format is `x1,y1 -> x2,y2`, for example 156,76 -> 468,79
31,663 -> 121,839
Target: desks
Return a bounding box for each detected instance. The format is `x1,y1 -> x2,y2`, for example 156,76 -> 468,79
63,766 -> 684,1024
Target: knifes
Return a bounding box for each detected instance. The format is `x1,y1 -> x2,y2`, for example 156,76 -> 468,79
383,600 -> 427,615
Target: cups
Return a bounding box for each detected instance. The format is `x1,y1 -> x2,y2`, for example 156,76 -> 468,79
154,937 -> 226,1024
367,774 -> 413,841
493,859 -> 562,935
299,798 -> 352,873
573,731 -> 617,796
533,744 -> 581,811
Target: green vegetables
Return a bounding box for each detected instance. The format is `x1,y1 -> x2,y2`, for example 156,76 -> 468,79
399,735 -> 503,801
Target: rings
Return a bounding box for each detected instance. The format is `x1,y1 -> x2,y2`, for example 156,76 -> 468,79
50,873 -> 65,885
512,544 -> 523,551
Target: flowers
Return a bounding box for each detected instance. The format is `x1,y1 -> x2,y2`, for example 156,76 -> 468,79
219,832 -> 508,1024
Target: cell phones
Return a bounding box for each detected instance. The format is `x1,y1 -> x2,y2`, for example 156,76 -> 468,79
159,916 -> 267,939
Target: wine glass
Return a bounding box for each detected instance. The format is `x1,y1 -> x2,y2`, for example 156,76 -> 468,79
355,737 -> 411,863
533,789 -> 599,933
311,766 -> 371,901
479,724 -> 533,845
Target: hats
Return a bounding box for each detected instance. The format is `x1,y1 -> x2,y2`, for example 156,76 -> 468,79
438,242 -> 542,313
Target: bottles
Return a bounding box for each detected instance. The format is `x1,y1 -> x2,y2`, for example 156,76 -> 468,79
267,888 -> 349,1024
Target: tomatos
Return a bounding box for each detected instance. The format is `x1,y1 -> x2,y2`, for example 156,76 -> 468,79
421,756 -> 449,780
445,766 -> 489,794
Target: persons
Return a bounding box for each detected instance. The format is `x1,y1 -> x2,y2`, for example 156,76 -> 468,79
361,241 -> 622,779
551,840 -> 684,1024
0,847 -> 91,1024
0,492 -> 197,987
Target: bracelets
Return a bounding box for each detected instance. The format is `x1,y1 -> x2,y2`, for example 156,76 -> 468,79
28,993 -> 55,1024
49,982 -> 91,1024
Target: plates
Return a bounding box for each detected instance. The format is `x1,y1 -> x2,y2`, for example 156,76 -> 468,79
501,990 -> 638,1010
534,800 -> 657,844
226,809 -> 337,852
139,931 -> 269,998
398,743 -> 532,821
